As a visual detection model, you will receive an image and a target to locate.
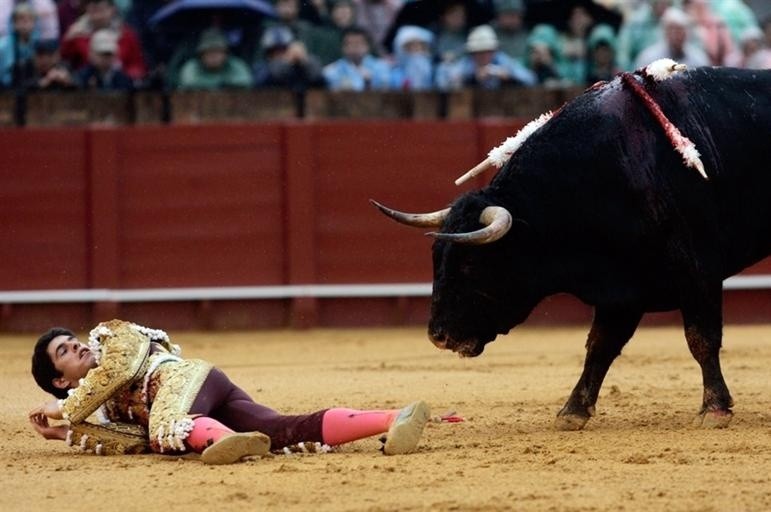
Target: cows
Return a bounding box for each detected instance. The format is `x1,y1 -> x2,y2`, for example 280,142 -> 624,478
365,63 -> 770,434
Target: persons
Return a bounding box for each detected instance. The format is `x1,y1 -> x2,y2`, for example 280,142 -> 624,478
30,319 -> 431,464
0,1 -> 770,87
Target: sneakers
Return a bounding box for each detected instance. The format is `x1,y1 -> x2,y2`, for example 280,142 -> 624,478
383,400 -> 431,456
198,430 -> 273,465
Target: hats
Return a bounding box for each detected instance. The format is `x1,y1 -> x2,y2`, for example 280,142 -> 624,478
661,7 -> 696,27
90,29 -> 119,57
261,25 -> 294,55
196,29 -> 227,53
466,24 -> 500,54
